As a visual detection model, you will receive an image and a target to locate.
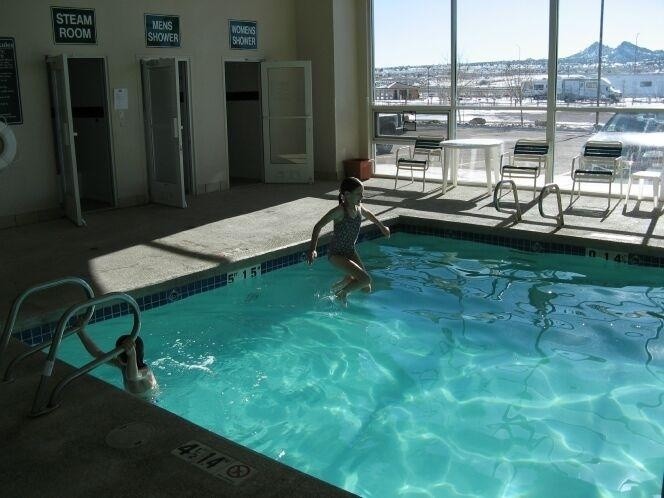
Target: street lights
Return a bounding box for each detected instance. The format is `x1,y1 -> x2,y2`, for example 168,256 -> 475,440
634,33 -> 641,73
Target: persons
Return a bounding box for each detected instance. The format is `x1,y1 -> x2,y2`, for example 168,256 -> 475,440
71,318 -> 161,407
304,177 -> 391,305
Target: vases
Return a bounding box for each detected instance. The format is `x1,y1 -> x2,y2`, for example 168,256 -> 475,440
343,158 -> 370,181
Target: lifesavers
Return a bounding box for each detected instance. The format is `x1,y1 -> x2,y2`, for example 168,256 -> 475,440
0,121 -> 16,170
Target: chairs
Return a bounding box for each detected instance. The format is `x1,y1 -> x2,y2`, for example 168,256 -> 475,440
565,139 -> 624,222
394,132 -> 445,194
493,137 -> 551,206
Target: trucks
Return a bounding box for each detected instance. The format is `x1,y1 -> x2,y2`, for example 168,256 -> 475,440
521,80 -> 562,100
561,77 -> 623,103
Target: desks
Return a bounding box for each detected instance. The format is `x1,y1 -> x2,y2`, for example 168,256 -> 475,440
439,138 -> 503,197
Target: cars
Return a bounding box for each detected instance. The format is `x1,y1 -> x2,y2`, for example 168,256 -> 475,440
579,112 -> 664,175
